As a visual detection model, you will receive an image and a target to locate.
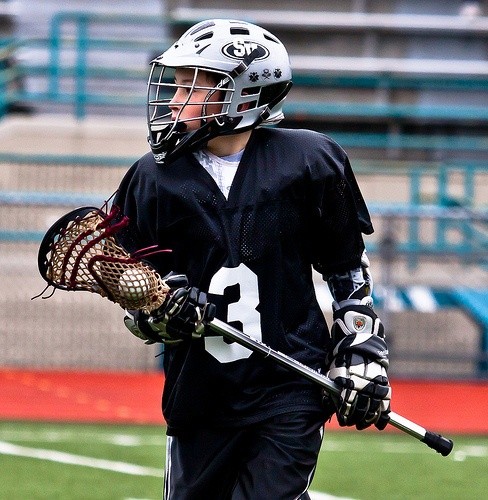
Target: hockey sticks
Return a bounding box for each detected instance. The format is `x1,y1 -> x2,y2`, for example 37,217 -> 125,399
36,206 -> 454,458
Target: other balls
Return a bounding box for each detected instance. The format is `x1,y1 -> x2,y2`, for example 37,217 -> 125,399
119,268 -> 149,300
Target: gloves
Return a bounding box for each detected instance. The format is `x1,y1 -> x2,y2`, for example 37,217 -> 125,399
121,274 -> 216,345
322,304 -> 392,431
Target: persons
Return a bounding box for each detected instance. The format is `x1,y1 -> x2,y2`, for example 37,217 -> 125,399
100,16 -> 391,500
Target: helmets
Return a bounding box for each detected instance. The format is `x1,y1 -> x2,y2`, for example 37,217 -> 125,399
144,19 -> 293,166
